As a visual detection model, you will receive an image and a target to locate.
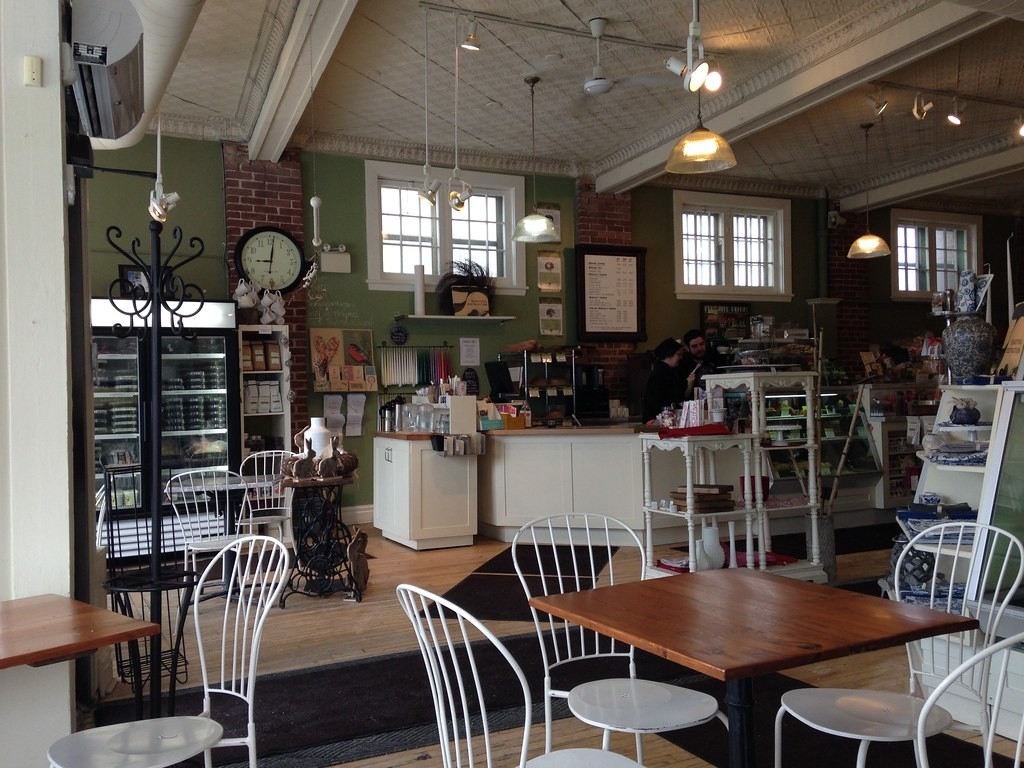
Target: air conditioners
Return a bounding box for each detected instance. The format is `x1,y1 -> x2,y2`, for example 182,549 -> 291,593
61,0 -> 146,138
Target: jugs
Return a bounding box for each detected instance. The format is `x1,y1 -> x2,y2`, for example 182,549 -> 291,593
375,396 -> 406,433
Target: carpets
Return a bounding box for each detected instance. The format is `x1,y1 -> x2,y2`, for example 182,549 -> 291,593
93,578 -> 894,768
471,544 -> 621,577
413,573 -> 599,623
653,668 -> 1024,767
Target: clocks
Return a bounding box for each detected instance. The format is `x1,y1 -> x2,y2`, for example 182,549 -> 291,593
234,224 -> 307,295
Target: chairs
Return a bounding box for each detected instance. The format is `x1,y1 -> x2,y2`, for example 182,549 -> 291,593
47,449 -> 1024,768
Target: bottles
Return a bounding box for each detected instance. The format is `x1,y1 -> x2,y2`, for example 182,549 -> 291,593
942,312 -> 996,377
520,403 -> 531,428
696,527 -> 724,570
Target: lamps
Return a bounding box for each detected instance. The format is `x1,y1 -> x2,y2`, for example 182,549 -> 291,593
321,244 -> 351,274
149,110 -> 181,221
417,0 -> 1024,260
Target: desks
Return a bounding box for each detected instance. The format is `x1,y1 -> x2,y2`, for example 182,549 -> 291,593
0,592 -> 163,671
527,567 -> 979,768
170,473 -> 284,608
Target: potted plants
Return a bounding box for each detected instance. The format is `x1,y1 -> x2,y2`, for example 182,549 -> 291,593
434,256 -> 496,316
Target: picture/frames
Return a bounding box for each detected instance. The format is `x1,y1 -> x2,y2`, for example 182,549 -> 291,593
119,263 -> 175,301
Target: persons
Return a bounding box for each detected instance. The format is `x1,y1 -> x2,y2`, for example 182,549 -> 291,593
683,330 -> 734,389
641,337 -> 695,425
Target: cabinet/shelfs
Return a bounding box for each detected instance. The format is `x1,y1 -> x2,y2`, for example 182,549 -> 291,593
371,433 -> 480,548
93,325 -> 294,565
279,474 -> 356,609
641,369 -> 1024,742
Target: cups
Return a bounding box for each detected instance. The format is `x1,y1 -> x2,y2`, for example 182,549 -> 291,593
609,399 -> 629,418
711,408 -> 726,423
402,403 -> 449,434
740,475 -> 769,504
548,420 -> 556,428
235,278 -> 287,325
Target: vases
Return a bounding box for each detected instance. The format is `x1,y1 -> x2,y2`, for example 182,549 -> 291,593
941,310 -> 998,377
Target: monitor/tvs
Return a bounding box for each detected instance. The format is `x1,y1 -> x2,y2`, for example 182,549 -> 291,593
485,361 -> 515,403
